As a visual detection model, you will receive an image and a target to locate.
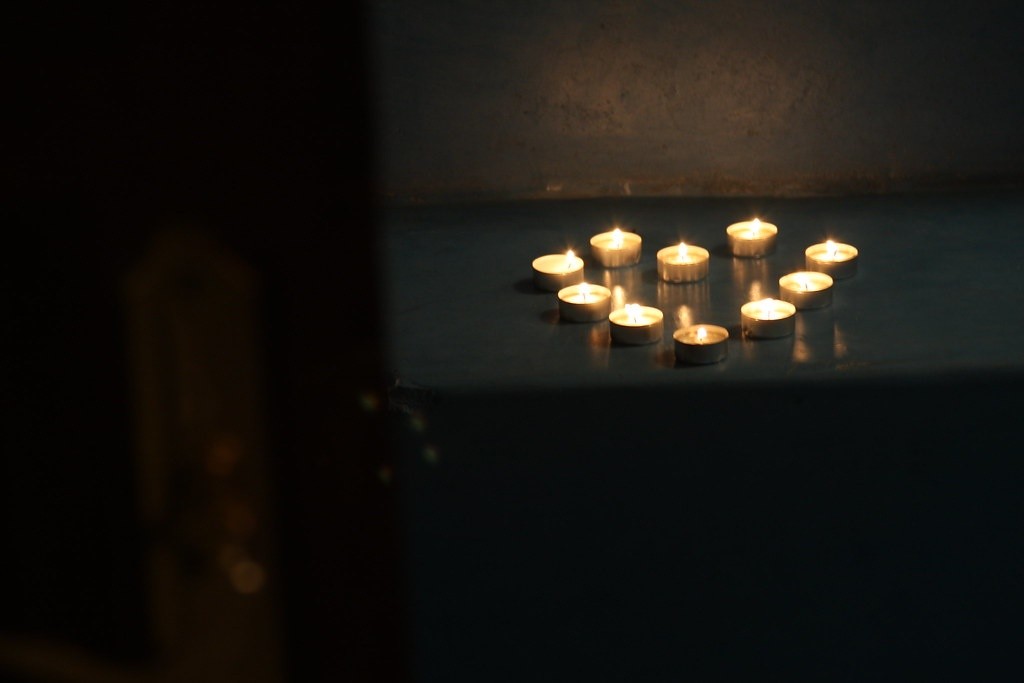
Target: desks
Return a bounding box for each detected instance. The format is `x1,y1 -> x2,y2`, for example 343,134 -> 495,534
376,181 -> 1024,683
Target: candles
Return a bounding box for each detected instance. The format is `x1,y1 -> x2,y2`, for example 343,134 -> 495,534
532,215 -> 860,366
